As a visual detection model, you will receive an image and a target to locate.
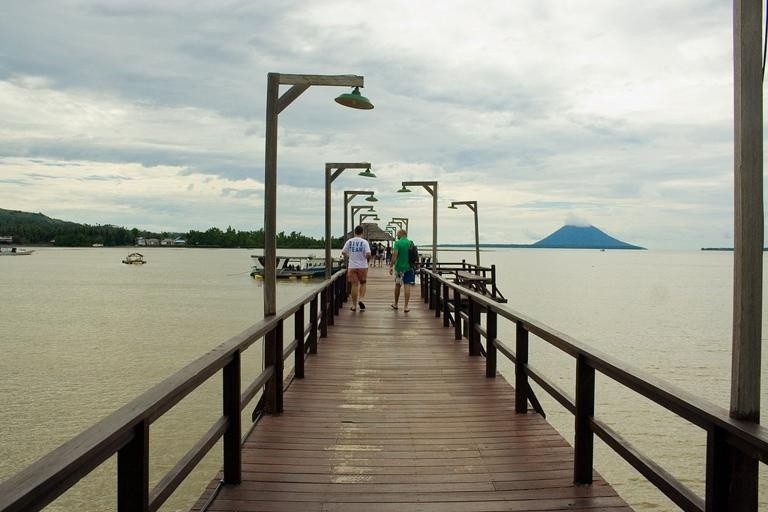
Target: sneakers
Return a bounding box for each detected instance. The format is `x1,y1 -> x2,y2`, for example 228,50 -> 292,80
350,301 -> 365,311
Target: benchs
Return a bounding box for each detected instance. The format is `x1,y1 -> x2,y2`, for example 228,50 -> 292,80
450,270 -> 487,295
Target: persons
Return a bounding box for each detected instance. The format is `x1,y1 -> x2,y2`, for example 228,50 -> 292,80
370,243 -> 377,267
341,226 -> 372,311
385,243 -> 391,265
377,243 -> 385,267
390,230 -> 414,311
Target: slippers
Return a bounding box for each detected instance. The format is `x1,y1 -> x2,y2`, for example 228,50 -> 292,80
404,309 -> 410,312
391,304 -> 398,309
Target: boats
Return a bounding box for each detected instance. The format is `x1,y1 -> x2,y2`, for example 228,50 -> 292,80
0,247 -> 36,255
249,255 -> 341,279
122,252 -> 147,265
92,243 -> 104,248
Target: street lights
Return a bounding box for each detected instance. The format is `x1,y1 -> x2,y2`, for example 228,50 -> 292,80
344,190 -> 380,245
325,163 -> 377,279
395,181 -> 438,273
263,72 -> 375,316
385,217 -> 409,246
447,200 -> 480,267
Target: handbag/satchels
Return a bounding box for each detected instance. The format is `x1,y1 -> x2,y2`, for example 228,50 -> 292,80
408,241 -> 419,264
